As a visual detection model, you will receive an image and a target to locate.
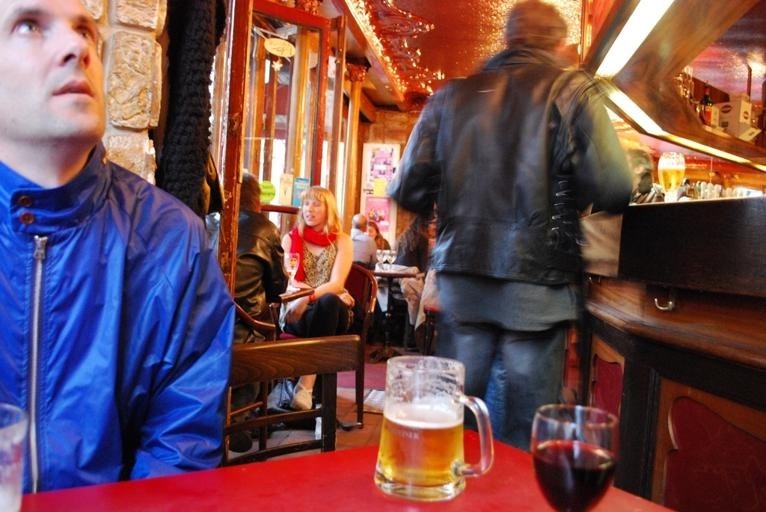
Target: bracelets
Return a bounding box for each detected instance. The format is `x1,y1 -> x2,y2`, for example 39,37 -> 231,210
308,290 -> 316,302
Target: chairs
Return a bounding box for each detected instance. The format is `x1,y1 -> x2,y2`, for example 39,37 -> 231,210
220,334 -> 361,464
345,263 -> 380,430
423,304 -> 441,355
232,303 -> 273,346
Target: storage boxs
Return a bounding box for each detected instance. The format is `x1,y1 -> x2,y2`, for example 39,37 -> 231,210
714,100 -> 751,135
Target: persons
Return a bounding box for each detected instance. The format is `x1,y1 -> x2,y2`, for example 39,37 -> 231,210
386,1 -> 634,455
280,186 -> 355,412
204,175 -> 287,453
627,148 -> 662,204
367,220 -> 391,254
368,209 -> 438,345
0,0 -> 237,491
351,214 -> 378,267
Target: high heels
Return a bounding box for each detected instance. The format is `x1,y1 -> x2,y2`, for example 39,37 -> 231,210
290,382 -> 313,410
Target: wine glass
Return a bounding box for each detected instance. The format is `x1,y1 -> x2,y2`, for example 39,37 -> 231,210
658,152 -> 685,203
285,253 -> 300,292
206,212 -> 221,234
375,249 -> 398,272
531,404 -> 619,512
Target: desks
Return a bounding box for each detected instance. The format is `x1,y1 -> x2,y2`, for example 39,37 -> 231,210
277,286 -> 317,309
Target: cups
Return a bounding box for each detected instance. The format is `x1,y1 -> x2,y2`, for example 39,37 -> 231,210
0,403 -> 27,512
373,356 -> 494,502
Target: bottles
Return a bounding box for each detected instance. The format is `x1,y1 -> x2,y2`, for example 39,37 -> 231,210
673,65 -> 713,127
682,178 -> 751,200
